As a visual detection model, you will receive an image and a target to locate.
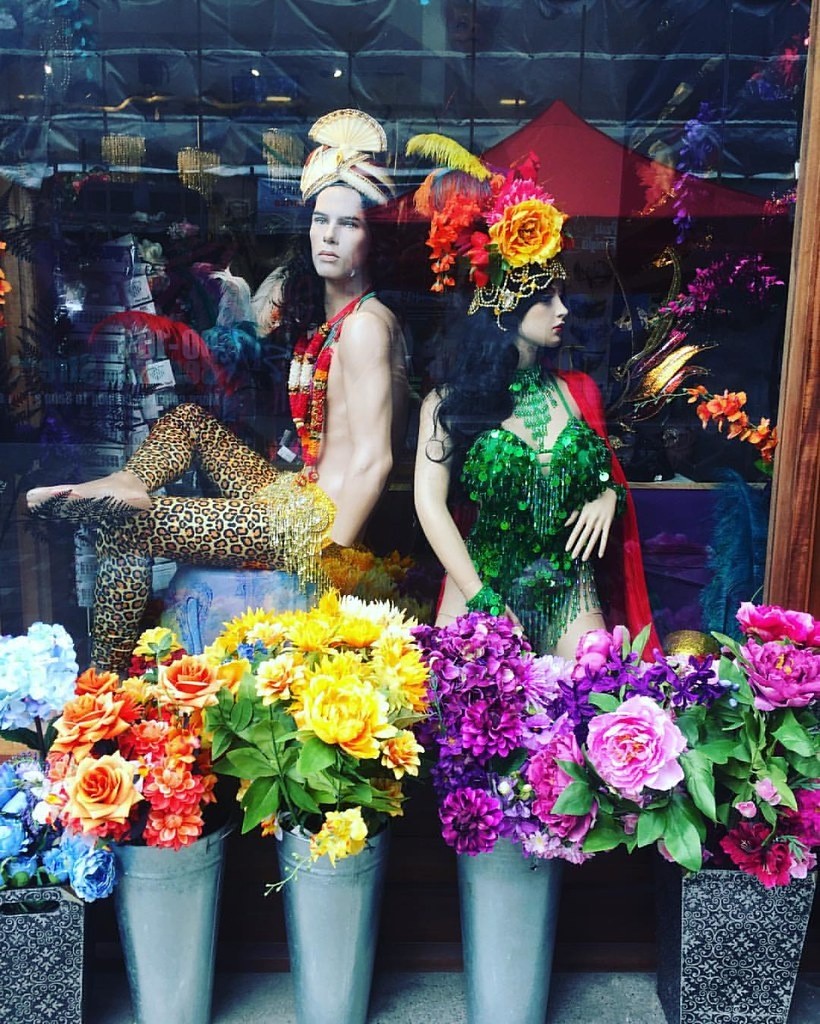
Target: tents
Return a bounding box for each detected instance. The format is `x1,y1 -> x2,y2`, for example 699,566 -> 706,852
365,99 -> 787,223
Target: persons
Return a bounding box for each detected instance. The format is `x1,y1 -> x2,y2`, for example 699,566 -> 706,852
26,108 -> 404,685
404,133 -> 663,674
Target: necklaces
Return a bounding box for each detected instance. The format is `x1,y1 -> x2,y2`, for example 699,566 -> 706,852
509,361 -> 557,450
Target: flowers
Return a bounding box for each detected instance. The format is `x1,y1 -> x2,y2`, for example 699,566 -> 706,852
606,162 -> 793,476
0,583 -> 820,904
404,133 -> 576,292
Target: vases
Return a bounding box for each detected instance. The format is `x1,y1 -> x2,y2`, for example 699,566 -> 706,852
276,819 -> 390,1024
657,850 -> 818,1024
0,887 -> 85,1024
457,835 -> 561,1024
114,817 -> 236,1024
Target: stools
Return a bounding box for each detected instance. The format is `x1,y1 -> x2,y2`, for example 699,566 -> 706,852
160,568 -> 314,662
